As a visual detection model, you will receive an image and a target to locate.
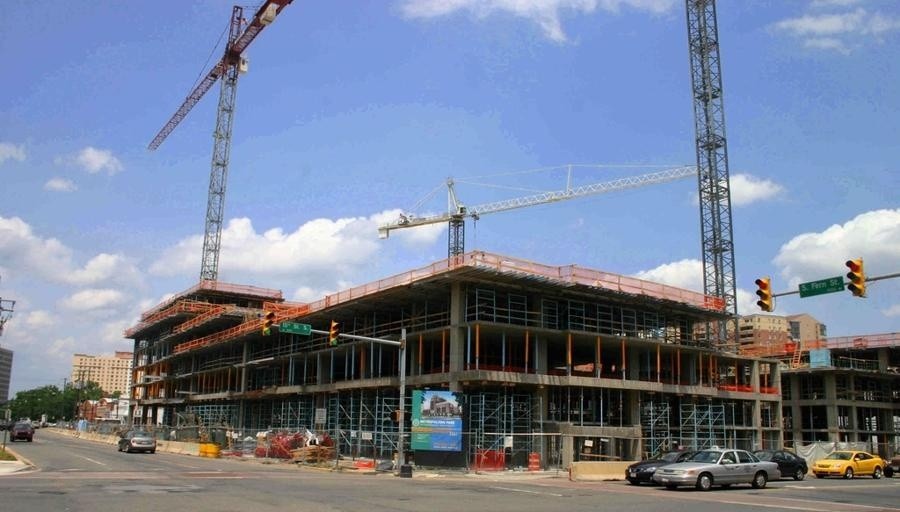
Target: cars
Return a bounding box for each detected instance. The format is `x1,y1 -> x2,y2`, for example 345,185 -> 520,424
624,445 -> 900,489
0,420 -> 73,441
118,430 -> 156,454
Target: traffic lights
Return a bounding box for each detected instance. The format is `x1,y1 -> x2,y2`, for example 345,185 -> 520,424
754,277 -> 772,312
845,257 -> 865,296
262,310 -> 274,337
329,320 -> 340,346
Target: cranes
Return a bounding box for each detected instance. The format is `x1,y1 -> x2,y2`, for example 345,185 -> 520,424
378,162 -> 699,271
145,0 -> 294,291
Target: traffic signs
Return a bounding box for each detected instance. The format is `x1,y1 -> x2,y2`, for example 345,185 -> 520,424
279,320 -> 312,336
798,274 -> 845,298
